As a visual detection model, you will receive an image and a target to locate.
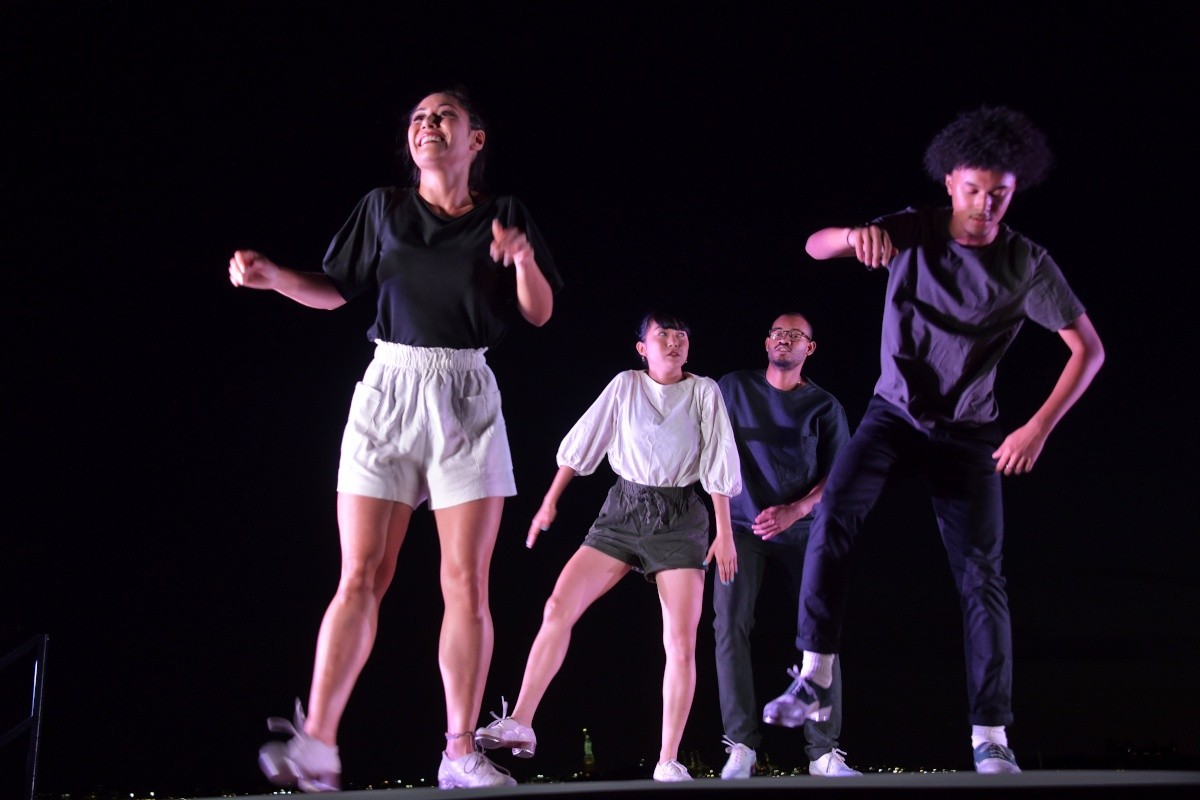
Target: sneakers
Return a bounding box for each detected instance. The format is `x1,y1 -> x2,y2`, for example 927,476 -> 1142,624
259,697 -> 342,792
721,734 -> 757,779
809,748 -> 863,777
438,750 -> 517,790
474,696 -> 537,758
763,663 -> 833,728
653,759 -> 696,783
973,741 -> 1022,775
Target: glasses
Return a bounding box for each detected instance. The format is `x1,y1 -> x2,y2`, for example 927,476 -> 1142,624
769,328 -> 811,342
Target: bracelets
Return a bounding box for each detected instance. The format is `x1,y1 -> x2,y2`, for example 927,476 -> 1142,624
841,226 -> 854,251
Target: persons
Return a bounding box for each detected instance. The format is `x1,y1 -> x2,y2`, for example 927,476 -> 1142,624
764,107 -> 1105,774
229,78 -> 559,792
484,303 -> 743,782
709,311 -> 860,778
